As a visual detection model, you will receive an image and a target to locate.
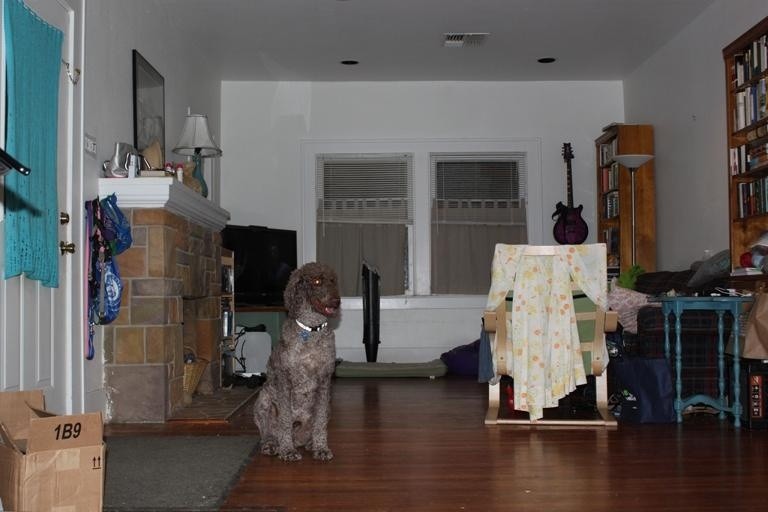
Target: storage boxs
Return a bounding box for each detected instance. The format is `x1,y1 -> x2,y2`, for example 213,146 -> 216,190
0,387 -> 108,511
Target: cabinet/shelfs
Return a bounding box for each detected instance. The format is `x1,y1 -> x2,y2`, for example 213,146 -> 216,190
721,16 -> 768,292
219,246 -> 237,392
594,124 -> 658,272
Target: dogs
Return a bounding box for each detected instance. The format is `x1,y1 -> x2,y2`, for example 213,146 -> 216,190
252,262 -> 341,461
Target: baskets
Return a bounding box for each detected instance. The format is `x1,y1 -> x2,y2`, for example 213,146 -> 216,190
183,343 -> 209,399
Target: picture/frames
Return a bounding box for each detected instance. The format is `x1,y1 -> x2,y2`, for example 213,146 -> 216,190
132,48 -> 166,170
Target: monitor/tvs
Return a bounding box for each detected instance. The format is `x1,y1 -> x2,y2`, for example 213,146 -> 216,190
221,225 -> 297,307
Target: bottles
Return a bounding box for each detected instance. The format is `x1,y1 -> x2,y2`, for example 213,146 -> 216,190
166,163 -> 172,172
176,163 -> 184,182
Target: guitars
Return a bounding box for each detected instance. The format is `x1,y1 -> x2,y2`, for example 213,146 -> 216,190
553,142 -> 588,244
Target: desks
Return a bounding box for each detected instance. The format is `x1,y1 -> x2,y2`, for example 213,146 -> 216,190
646,295 -> 755,429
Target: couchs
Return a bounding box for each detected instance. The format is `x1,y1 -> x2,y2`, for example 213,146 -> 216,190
607,269 -> 735,421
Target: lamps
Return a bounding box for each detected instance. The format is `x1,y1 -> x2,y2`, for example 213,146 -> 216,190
612,154 -> 656,265
172,112 -> 223,198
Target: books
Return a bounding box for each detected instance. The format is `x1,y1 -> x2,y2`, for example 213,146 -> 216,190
729,35 -> 768,224
728,272 -> 764,276
596,134 -> 625,268
734,266 -> 758,272
602,122 -> 623,132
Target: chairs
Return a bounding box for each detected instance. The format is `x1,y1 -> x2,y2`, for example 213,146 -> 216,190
480,242 -> 618,429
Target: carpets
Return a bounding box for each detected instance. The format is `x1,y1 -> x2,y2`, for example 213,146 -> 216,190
104,434 -> 261,512
